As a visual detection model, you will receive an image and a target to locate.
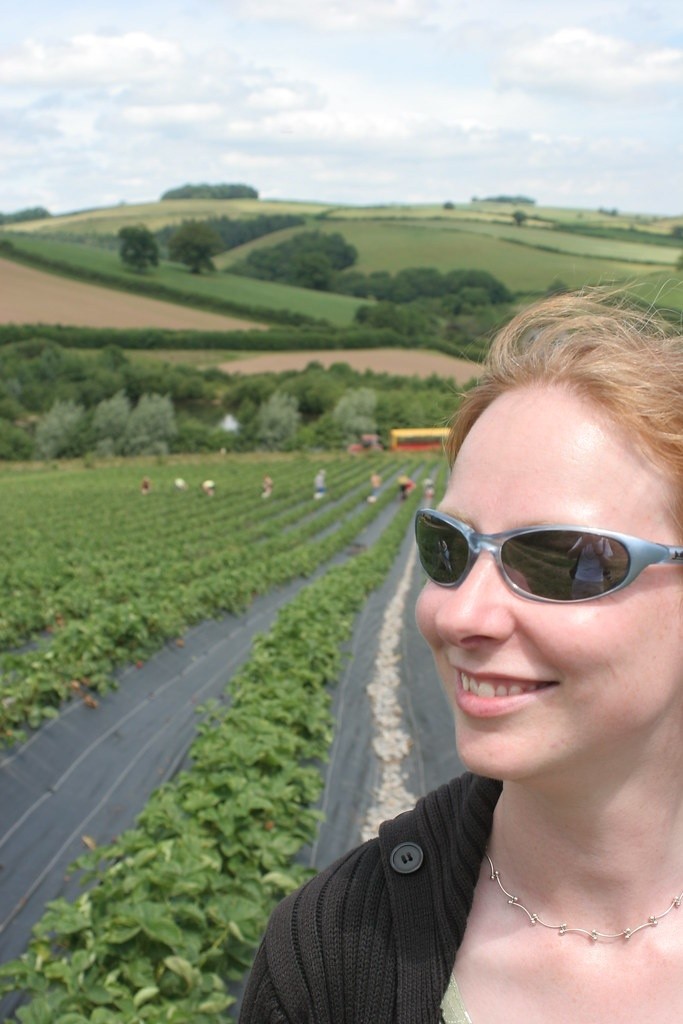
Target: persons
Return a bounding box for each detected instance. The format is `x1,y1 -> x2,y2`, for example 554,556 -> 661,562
438,537 -> 452,573
202,480 -> 214,495
422,478 -> 435,497
261,475 -> 272,497
397,475 -> 414,499
141,476 -> 150,494
235,291 -> 683,1024
313,469 -> 326,494
567,533 -> 613,600
370,472 -> 381,494
175,477 -> 185,491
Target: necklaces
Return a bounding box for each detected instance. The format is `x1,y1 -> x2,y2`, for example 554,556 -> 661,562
584,546 -> 596,560
485,845 -> 683,940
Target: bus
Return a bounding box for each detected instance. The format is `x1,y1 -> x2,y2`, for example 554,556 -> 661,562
392,427 -> 452,450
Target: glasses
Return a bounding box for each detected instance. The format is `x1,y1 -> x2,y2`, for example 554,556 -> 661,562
415,508 -> 683,604
